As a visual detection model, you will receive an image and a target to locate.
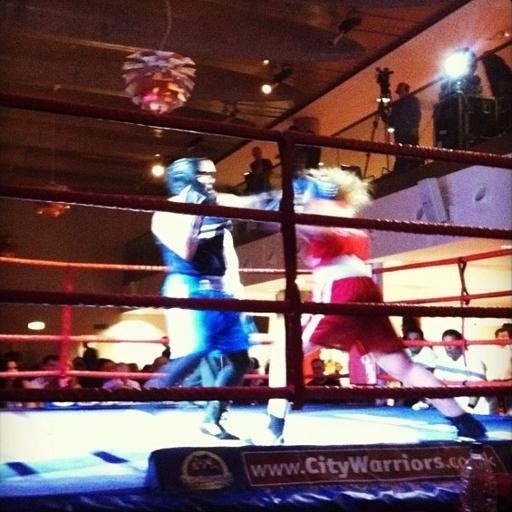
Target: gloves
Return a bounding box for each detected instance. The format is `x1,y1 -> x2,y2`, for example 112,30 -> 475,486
291,178 -> 319,206
185,184 -> 217,229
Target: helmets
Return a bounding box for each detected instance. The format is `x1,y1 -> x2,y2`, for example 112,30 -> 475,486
167,158 -> 217,193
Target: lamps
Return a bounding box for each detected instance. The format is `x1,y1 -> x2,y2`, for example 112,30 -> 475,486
327,18 -> 361,45
261,72 -> 285,93
121,0 -> 199,118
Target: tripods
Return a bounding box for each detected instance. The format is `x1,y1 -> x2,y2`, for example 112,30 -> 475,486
363,93 -> 393,179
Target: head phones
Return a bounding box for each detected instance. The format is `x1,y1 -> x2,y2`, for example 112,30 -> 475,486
396,84 -> 409,94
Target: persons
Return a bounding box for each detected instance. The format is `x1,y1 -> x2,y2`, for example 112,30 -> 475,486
139,157 -> 339,444
186,357 -> 342,387
277,126 -> 324,177
1,336 -> 170,389
246,145 -> 276,196
400,322 -> 512,417
202,165 -> 488,443
379,81 -> 422,169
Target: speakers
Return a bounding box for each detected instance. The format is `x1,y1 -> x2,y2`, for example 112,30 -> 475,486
433,94 -> 498,150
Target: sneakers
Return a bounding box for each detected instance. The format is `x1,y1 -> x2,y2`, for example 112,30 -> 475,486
201,419 -> 241,441
242,430 -> 285,446
